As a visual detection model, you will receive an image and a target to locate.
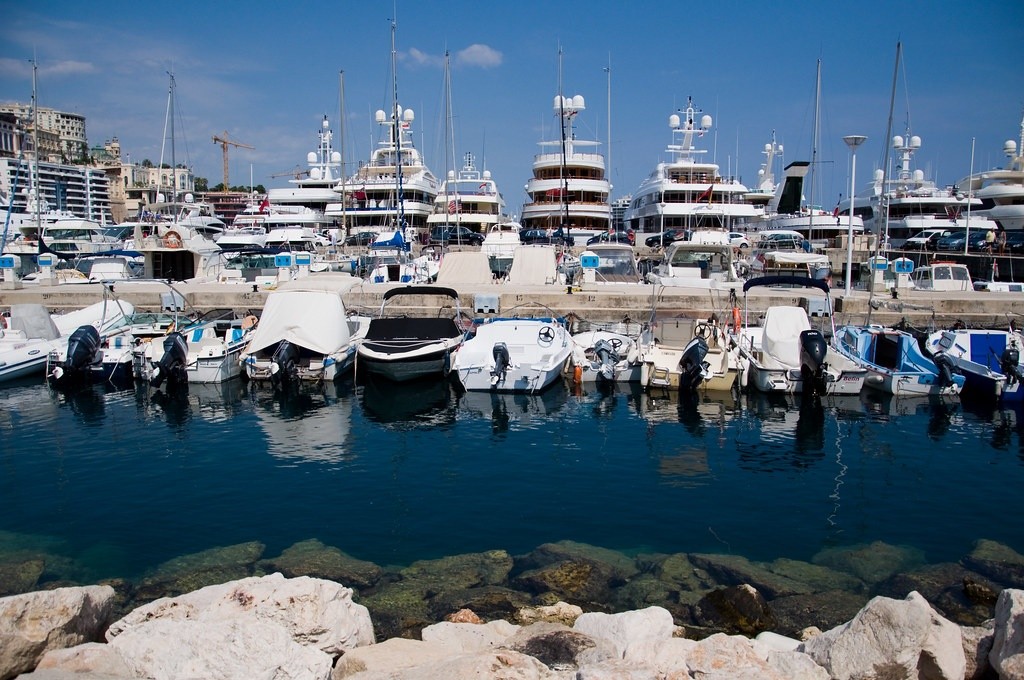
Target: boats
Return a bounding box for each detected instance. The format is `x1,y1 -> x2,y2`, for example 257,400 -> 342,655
237,270 -> 372,407
559,283 -> 668,385
45,277 -> 205,406
924,315 -> 1024,408
727,274 -> 867,397
352,282 -> 468,383
452,299 -> 576,397
834,322 -> 967,400
0,297 -> 138,389
126,279 -> 263,386
640,311 -> 754,392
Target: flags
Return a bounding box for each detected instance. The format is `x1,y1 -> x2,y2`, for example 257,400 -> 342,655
698,185 -> 712,198
448,199 -> 461,214
259,197 -> 269,212
833,200 -> 840,217
350,187 -> 366,200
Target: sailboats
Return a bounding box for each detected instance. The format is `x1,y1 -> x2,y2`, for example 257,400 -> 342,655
1,0 -> 1023,332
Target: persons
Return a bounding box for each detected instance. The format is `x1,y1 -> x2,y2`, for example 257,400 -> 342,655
996,227 -> 1007,256
986,228 -> 996,254
879,228 -> 884,246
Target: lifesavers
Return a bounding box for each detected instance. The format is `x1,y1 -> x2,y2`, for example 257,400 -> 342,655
733,308 -> 742,335
163,230 -> 183,248
